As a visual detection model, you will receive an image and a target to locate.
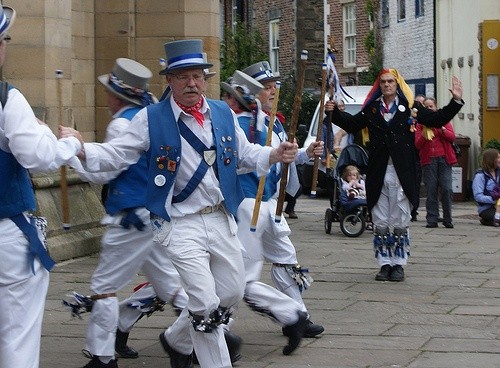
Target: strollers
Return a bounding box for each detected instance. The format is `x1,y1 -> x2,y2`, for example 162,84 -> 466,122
324,144 -> 372,237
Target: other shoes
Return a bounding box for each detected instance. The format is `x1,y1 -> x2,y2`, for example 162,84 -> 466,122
77,350 -> 119,368
285,207 -> 298,219
158,331 -> 194,368
115,326 -> 139,358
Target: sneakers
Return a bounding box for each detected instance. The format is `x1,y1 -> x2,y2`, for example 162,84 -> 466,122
282,310 -> 307,354
390,265 -> 404,281
282,320 -> 324,337
376,264 -> 392,280
442,223 -> 453,228
427,223 -> 438,228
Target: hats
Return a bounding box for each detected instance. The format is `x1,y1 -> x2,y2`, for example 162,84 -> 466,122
201,52 -> 216,77
97,58 -> 160,107
220,70 -> 265,113
0,0 -> 17,34
159,39 -> 214,75
242,61 -> 284,83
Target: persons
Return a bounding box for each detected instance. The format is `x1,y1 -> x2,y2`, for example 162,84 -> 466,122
473,148 -> 500,227
410,95 -> 426,220
414,97 -> 457,228
221,69 -> 308,352
333,128 -> 370,151
341,165 -> 367,200
325,68 -> 465,281
80,58 -> 241,368
283,126 -> 302,218
115,281 -> 242,365
59,38 -> 299,368
241,61 -> 325,337
0,3 -> 86,368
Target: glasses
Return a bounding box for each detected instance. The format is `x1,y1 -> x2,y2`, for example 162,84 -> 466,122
171,72 -> 206,81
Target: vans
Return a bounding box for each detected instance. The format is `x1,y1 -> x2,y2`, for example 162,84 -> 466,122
296,86 -> 373,196
